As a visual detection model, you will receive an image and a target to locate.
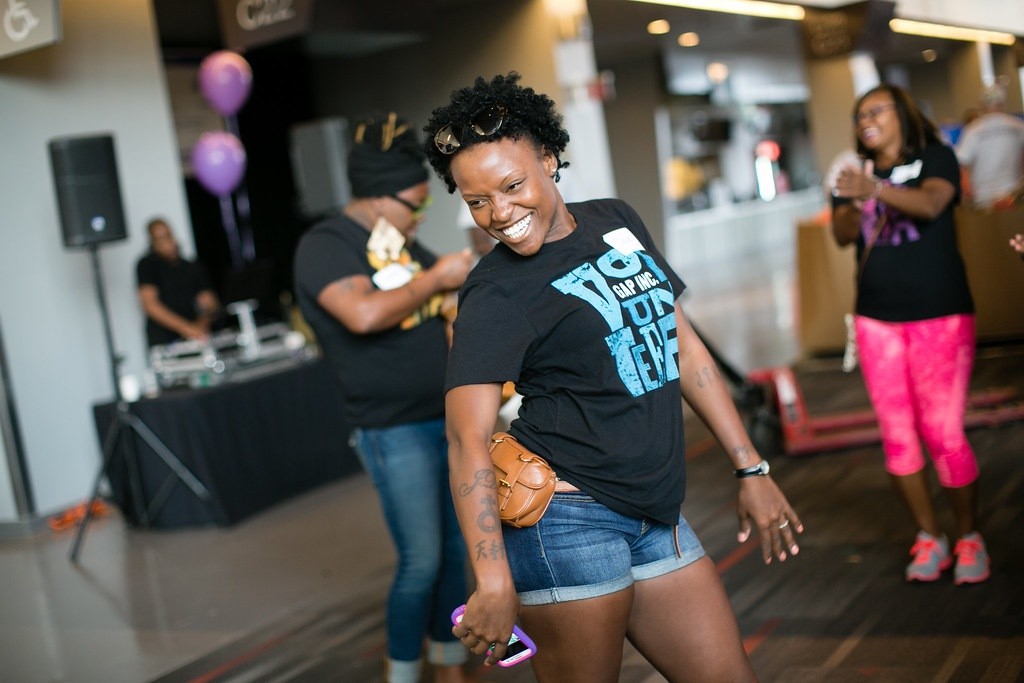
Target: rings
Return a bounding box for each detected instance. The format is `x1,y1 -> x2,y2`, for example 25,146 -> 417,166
779,519 -> 789,529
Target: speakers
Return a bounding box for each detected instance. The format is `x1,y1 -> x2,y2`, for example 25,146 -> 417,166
290,119 -> 351,219
48,131 -> 128,246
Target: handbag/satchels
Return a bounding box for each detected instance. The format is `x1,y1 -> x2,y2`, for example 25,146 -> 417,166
487,432 -> 580,527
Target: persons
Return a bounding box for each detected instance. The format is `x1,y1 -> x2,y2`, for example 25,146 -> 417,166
420,69 -> 805,683
135,219 -> 219,346
941,85 -> 1024,210
822,84 -> 990,585
293,111 -> 473,682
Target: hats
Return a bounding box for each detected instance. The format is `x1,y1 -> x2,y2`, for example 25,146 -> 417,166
347,111 -> 428,200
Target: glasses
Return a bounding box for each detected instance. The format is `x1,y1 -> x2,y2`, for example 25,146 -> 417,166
854,104 -> 900,123
391,194 -> 430,219
435,103 -> 510,154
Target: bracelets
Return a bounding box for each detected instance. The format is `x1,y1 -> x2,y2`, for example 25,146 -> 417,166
859,174 -> 883,200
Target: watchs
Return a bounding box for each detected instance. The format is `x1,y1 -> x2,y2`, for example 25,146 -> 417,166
733,460 -> 771,479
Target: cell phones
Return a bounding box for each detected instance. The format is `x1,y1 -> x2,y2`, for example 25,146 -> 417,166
451,605 -> 537,667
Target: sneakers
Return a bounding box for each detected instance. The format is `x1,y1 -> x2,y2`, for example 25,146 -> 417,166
955,533 -> 991,584
905,530 -> 951,581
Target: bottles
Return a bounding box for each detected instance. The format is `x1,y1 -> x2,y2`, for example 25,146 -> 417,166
188,370 -> 226,388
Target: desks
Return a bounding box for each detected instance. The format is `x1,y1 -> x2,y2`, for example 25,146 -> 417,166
91,346 -> 368,528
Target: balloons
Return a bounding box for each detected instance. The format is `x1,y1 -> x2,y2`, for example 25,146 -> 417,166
201,51 -> 249,116
195,128 -> 245,195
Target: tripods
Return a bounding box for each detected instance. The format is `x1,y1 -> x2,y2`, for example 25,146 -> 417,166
65,243 -> 231,561
